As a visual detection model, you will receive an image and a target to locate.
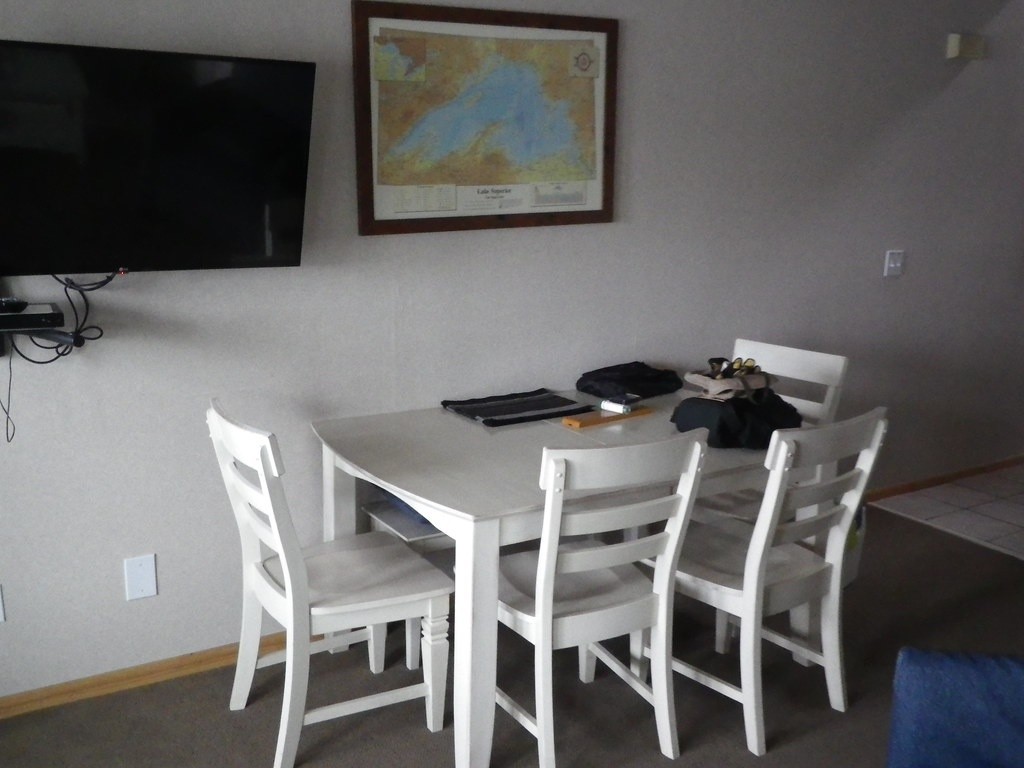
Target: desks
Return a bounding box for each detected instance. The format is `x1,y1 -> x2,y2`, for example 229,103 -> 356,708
311,385 -> 827,766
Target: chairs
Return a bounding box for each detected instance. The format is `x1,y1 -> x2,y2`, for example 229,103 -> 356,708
452,424 -> 713,767
628,334 -> 898,754
205,397 -> 457,768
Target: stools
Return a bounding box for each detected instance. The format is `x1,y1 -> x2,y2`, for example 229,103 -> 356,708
362,495 -> 455,671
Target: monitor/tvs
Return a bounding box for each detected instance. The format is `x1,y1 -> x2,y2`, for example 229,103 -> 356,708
0,39 -> 316,277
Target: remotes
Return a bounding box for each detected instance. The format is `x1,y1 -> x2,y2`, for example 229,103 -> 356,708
0,297 -> 28,314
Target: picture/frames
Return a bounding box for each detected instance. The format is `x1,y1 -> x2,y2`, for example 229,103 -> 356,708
351,2 -> 620,236
